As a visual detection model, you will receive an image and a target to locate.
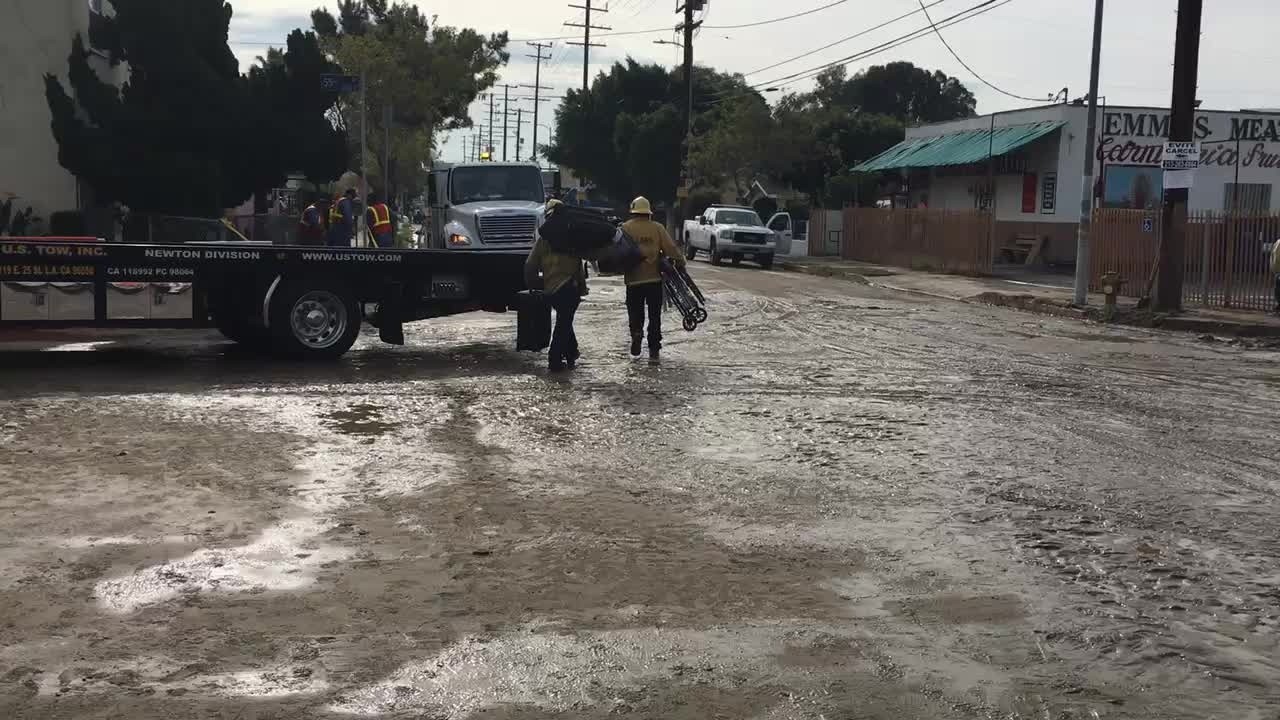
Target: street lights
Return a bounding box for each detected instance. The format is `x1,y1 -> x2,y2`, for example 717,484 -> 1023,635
653,40 -> 694,245
359,79 -> 383,247
537,123 -> 552,169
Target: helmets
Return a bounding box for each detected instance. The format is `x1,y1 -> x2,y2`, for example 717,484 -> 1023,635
544,199 -> 564,219
629,196 -> 653,214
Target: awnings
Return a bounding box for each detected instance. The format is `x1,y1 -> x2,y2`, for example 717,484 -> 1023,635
848,119 -> 1069,176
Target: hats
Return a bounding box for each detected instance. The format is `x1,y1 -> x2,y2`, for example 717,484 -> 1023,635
347,189 -> 357,196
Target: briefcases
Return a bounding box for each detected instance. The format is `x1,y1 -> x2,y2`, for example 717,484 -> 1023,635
517,288 -> 551,352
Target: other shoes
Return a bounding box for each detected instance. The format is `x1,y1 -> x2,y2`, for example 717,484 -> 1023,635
631,329 -> 642,355
651,350 -> 659,358
566,349 -> 580,364
550,361 -> 565,369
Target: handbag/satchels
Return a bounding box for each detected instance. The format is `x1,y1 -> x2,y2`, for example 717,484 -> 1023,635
597,227 -> 640,273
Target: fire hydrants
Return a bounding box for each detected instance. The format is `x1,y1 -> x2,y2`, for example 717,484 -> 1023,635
1100,271 -> 1129,306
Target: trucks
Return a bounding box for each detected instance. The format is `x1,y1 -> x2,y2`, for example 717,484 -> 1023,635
426,161 -> 545,250
540,167 -> 563,199
0,234 -> 529,363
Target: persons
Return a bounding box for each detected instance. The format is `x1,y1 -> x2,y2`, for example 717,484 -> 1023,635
300,200 -> 321,224
327,188 -> 357,247
524,198 -> 589,371
391,201 -> 399,211
618,196 -> 686,358
366,193 -> 394,248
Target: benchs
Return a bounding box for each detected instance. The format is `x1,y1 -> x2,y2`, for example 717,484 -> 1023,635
999,233 -> 1048,265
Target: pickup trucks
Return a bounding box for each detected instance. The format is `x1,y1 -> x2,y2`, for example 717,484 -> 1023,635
683,203 -> 793,267
